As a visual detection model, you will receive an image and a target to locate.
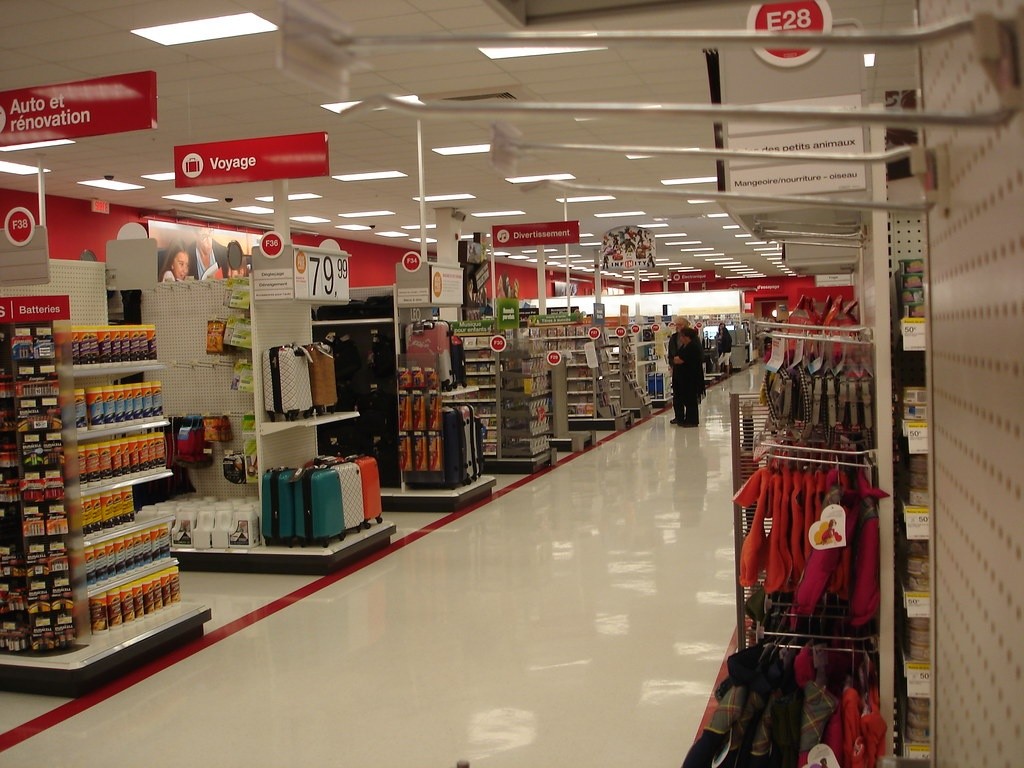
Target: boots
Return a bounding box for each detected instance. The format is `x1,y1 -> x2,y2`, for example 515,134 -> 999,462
724,365 -> 729,379
716,365 -> 721,374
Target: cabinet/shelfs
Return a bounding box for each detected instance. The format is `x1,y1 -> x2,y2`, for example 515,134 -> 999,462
56,285 -> 756,697
894,316 -> 931,761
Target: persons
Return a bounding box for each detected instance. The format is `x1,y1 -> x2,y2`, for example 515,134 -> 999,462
669,318 -> 690,426
717,327 -> 732,377
188,226 -> 228,280
158,239 -> 194,282
674,327 -> 707,427
216,241 -> 252,278
716,323 -> 725,373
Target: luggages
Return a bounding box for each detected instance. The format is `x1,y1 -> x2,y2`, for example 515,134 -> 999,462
262,342 -> 338,422
403,319 -> 468,392
261,453 -> 384,547
403,404 -> 484,491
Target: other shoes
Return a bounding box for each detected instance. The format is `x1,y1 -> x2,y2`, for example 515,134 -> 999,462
676,419 -> 699,428
669,418 -> 685,424
